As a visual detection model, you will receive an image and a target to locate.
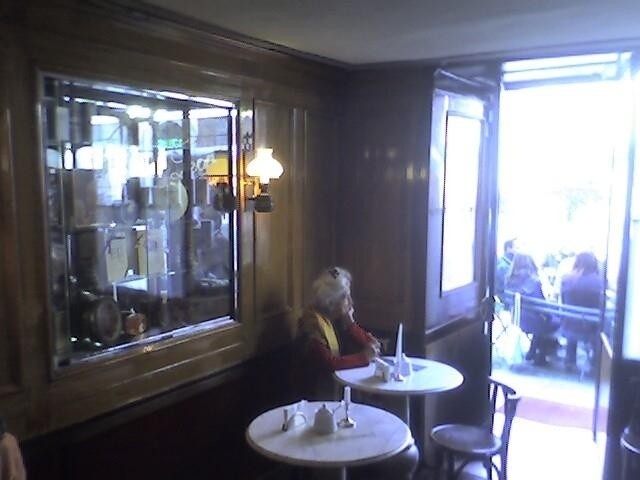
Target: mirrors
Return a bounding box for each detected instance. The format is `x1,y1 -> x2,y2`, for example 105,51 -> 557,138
33,69 -> 242,382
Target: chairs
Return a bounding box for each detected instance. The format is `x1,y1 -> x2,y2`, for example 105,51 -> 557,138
427,375 -> 522,480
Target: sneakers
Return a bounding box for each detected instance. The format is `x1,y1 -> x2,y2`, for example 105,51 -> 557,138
525,351 -> 546,366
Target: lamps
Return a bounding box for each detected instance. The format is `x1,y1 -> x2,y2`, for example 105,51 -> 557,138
244,148 -> 286,214
202,150 -> 233,213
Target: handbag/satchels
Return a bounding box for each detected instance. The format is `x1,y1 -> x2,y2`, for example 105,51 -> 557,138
501,325 -> 521,365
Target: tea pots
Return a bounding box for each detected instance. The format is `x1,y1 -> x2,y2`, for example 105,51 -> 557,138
313,403 -> 339,434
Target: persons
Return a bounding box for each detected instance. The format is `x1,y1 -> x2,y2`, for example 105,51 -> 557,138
503,253 -> 561,365
289,266 -> 382,401
495,239 -> 519,311
560,249 -> 603,377
